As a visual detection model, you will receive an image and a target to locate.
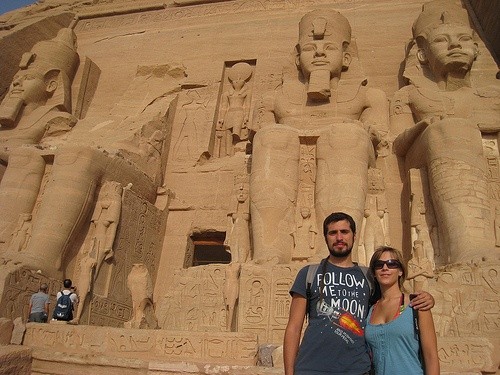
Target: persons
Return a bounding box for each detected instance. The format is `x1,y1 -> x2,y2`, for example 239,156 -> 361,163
407,195 -> 439,293
390,1 -> 500,274
27,283 -> 50,323
220,63 -> 253,157
0,27 -> 156,324
365,246 -> 440,375
250,8 -> 387,265
283,211 -> 435,375
363,182 -> 389,267
224,173 -> 251,264
290,208 -> 317,264
53,279 -> 79,321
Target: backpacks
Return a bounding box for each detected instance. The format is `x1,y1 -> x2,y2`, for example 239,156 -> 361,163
52,291 -> 74,321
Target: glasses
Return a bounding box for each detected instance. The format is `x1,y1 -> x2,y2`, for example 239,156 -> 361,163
374,260 -> 398,269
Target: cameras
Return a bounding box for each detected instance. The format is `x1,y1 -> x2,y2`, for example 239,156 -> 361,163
71,287 -> 74,289
